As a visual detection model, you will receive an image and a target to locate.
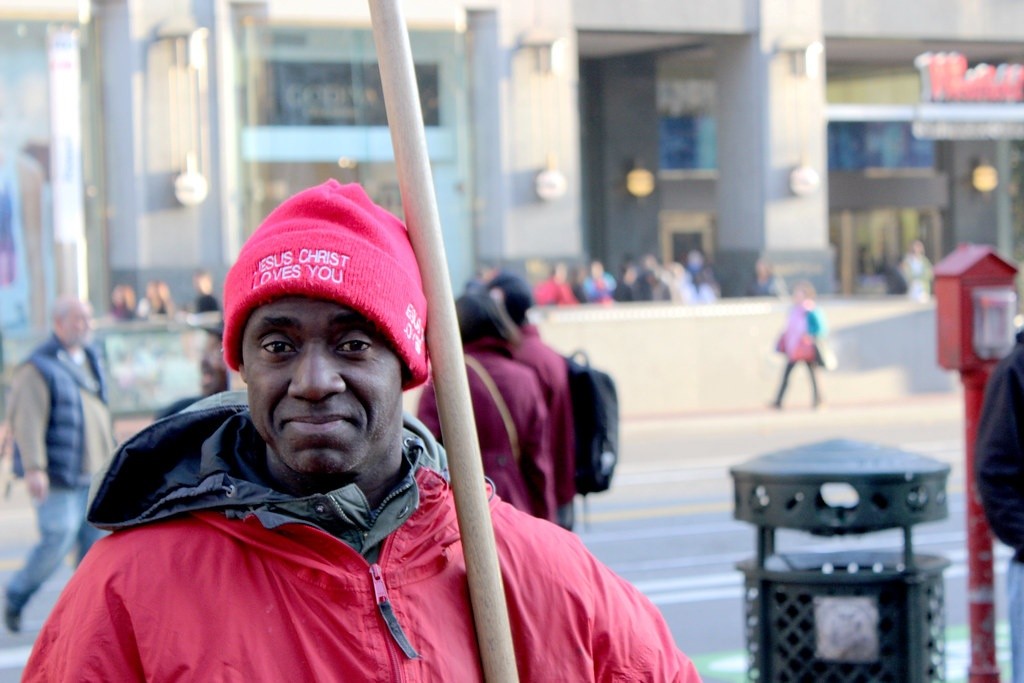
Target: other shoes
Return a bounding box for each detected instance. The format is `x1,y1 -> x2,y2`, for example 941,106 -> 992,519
4,594 -> 20,631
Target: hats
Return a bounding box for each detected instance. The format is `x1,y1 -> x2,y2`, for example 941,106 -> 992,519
223,179 -> 429,392
466,273 -> 532,316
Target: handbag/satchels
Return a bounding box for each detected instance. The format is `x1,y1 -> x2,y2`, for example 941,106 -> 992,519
793,335 -> 815,360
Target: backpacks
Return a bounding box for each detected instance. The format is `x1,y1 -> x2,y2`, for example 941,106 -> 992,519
562,350 -> 619,496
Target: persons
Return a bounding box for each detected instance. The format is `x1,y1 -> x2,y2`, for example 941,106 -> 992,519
973,326 -> 1024,683
20,177 -> 701,683
419,274 -> 576,531
771,282 -> 824,409
105,270 -> 219,320
534,248 -> 721,306
860,239 -> 934,297
0,298 -> 120,631
465,263 -> 497,297
748,258 -> 785,297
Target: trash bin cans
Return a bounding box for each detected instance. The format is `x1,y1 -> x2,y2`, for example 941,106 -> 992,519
730,436 -> 952,683
